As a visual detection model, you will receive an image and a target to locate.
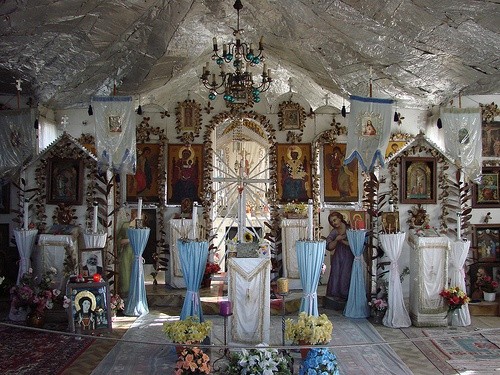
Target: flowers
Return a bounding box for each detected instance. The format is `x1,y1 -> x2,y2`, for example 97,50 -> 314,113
161,234 -> 340,374
284,200 -> 321,217
368,266 -> 497,312
12,267 -> 71,317
479,102 -> 500,124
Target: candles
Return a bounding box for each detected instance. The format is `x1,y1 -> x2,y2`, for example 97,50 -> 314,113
220,301 -> 230,314
457,212 -> 461,241
93,201 -> 98,234
389,200 -> 393,212
277,278 -> 288,293
137,196 -> 143,219
24,198 -> 29,231
308,199 -> 314,241
192,201 -> 199,239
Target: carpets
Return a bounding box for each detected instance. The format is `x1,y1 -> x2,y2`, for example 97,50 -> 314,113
400,326 -> 500,375
0,315 -> 100,375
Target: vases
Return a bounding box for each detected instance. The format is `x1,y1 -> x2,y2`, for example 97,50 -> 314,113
373,311 -> 384,324
287,213 -> 307,219
298,339 -> 313,356
202,277 -> 211,287
482,291 -> 496,302
29,314 -> 42,327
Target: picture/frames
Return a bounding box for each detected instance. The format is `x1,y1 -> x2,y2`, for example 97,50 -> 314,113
350,210 -> 400,233
47,104 -> 205,206
69,282 -> 112,335
80,248 -> 106,282
401,158 -> 500,301
276,107 -> 358,204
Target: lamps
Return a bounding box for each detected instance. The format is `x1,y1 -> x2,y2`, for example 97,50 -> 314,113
201,0 -> 273,109
